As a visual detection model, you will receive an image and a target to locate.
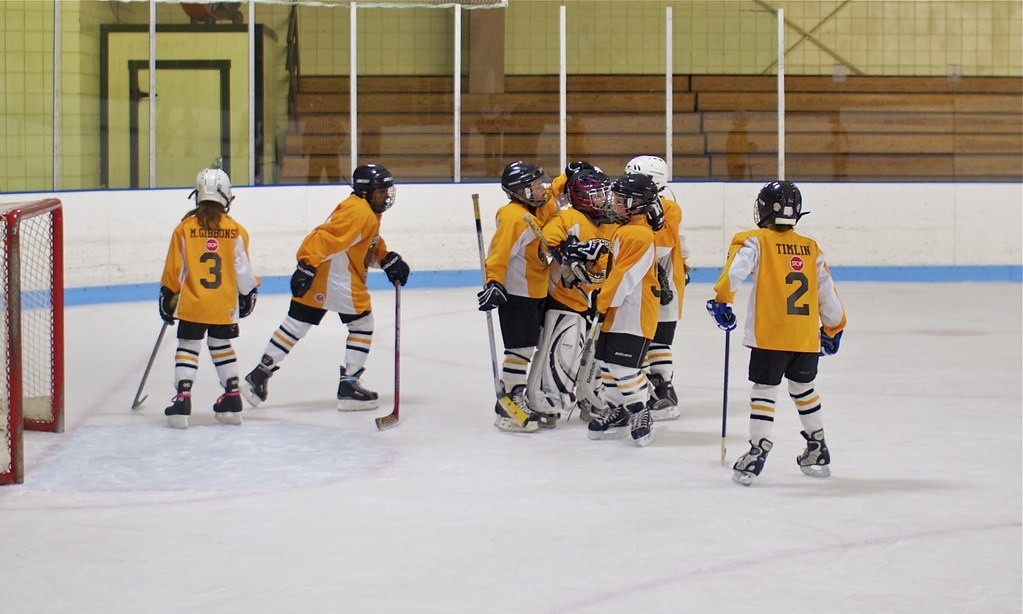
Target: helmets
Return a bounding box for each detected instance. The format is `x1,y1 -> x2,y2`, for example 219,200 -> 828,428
566,167 -> 610,213
193,168 -> 232,208
501,162 -> 551,206
352,163 -> 395,212
752,180 -> 802,226
623,155 -> 667,193
606,175 -> 657,224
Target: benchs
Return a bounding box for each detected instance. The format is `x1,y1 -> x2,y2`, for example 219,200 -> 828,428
276,73 -> 1023,190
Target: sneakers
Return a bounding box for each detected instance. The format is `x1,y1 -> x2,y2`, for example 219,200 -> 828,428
164,379 -> 193,428
495,371 -> 680,446
213,377 -> 243,425
337,366 -> 378,410
796,429 -> 831,476
732,439 -> 772,484
238,354 -> 280,407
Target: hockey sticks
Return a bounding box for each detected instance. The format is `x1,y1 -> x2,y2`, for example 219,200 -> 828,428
471,193 -> 529,428
578,310 -> 608,410
720,306 -> 734,465
131,294 -> 178,411
374,280 -> 404,432
523,213 -> 589,303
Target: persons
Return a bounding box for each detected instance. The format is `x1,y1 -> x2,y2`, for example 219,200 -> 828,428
159,168 -> 267,430
240,164 -> 411,412
476,155 -> 692,447
706,181 -> 847,487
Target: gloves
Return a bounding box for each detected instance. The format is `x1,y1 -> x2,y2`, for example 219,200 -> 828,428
290,261 -> 317,297
563,240 -> 605,260
238,289 -> 258,318
158,286 -> 176,325
706,299 -> 737,332
475,283 -> 505,311
644,198 -> 665,229
380,251 -> 409,287
818,326 -> 842,356
564,160 -> 601,180
